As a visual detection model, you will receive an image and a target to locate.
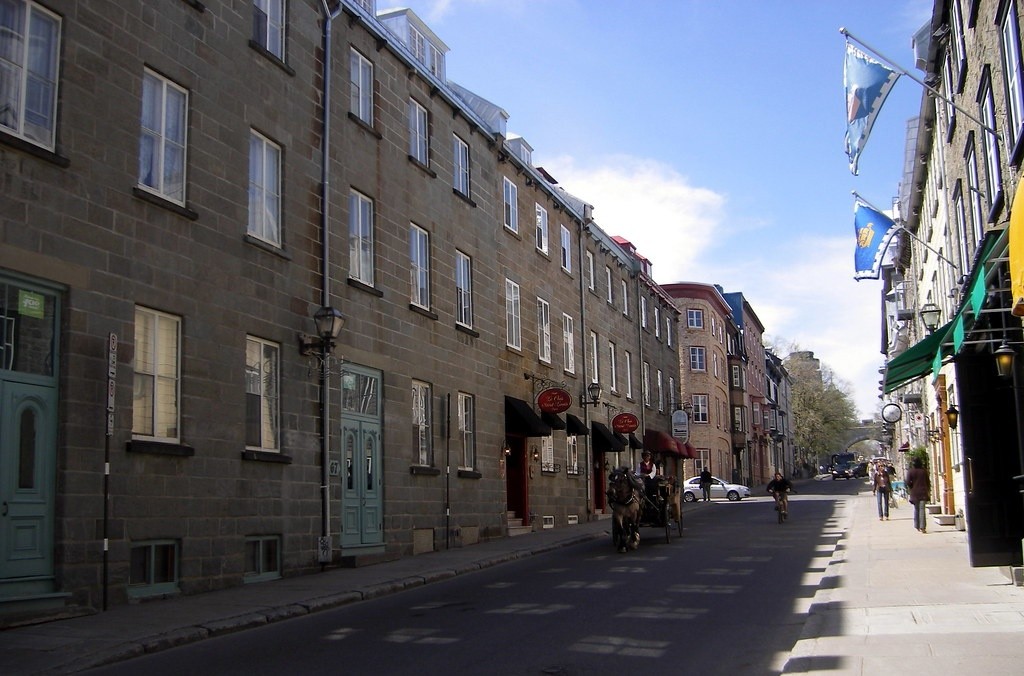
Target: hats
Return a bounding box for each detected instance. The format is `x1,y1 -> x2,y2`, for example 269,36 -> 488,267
641,449 -> 651,454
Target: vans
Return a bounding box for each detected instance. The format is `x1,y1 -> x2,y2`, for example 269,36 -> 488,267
848,463 -> 867,479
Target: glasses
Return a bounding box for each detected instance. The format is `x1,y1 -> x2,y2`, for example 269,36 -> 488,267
878,465 -> 884,468
644,454 -> 651,457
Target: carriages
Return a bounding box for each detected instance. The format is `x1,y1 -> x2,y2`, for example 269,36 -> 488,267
607,465 -> 684,554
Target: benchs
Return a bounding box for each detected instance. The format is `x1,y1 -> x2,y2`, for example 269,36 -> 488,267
645,483 -> 671,498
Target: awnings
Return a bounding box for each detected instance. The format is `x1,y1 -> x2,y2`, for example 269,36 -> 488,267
878,224 -> 1010,400
592,420 -> 628,452
505,395 -> 565,437
566,413 -> 589,435
629,433 -> 644,449
645,430 -> 697,461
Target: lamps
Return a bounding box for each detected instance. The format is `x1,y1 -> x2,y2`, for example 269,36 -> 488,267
920,154 -> 929,164
530,443 -> 539,464
931,24 -> 951,45
912,208 -> 919,216
917,184 -> 923,193
943,404 -> 960,431
993,334 -> 1024,377
299,308 -> 346,356
501,440 -> 511,458
593,457 -> 599,471
926,79 -> 942,97
605,457 -> 610,472
919,304 -> 942,337
684,402 -> 693,419
923,119 -> 934,132
580,383 -> 603,408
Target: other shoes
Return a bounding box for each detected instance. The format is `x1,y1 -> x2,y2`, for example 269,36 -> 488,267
885,515 -> 888,520
879,515 -> 883,521
784,513 -> 788,519
708,500 -> 710,503
657,496 -> 664,501
701,501 -> 706,503
775,505 -> 779,510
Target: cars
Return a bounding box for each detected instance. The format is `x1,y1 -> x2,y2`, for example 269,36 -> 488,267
683,476 -> 750,502
832,465 -> 849,481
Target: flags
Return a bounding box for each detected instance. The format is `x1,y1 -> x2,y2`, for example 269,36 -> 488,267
854,198 -> 902,280
843,38 -> 901,177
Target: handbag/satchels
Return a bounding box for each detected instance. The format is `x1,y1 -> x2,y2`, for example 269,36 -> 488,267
889,497 -> 898,509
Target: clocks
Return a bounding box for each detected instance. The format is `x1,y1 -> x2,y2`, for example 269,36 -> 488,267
882,403 -> 904,424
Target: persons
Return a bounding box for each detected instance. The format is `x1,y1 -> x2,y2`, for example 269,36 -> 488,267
873,463 -> 893,520
636,450 -> 664,503
767,472 -> 791,519
907,457 -> 930,533
867,460 -> 896,482
701,467 -> 711,502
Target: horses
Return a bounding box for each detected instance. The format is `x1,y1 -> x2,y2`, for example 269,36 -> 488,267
607,466 -> 645,553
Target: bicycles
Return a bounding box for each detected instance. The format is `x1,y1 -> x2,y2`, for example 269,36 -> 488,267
772,491 -> 789,524
